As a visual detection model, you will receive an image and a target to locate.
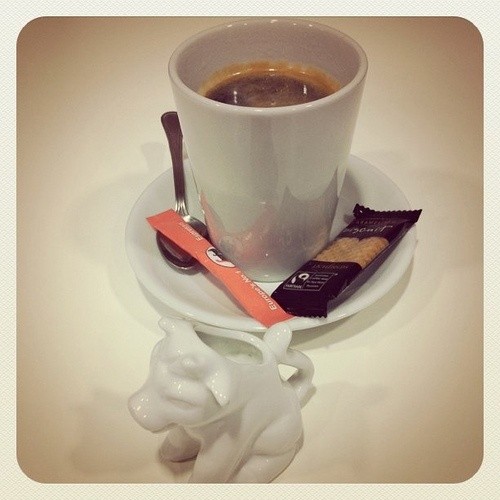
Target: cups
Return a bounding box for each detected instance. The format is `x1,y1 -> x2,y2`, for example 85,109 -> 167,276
167,19 -> 368,282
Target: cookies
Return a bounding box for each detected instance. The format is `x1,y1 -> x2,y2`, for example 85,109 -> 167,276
311,235 -> 390,269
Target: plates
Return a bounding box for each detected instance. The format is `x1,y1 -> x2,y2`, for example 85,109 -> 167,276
123,149 -> 420,332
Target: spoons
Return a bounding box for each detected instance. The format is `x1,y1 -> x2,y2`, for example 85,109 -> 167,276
156,111 -> 208,269
